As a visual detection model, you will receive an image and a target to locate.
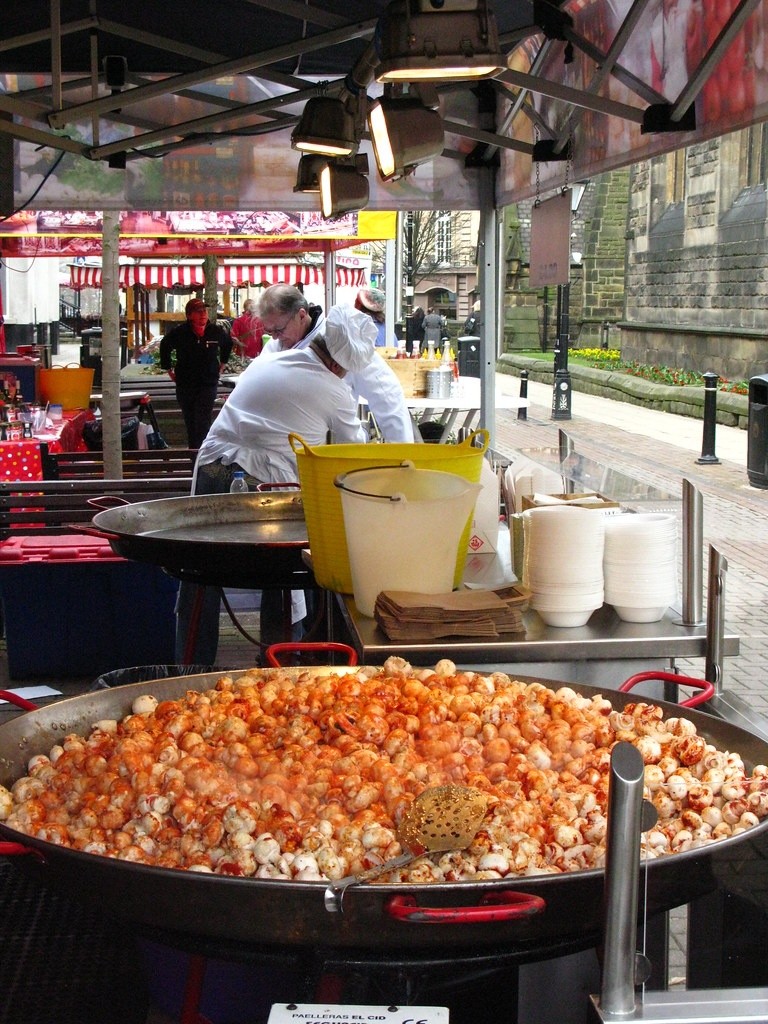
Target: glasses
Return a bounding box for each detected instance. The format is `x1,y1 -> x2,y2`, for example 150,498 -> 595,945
263,314 -> 293,336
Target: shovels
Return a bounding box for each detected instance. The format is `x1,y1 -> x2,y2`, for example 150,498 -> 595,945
325,785 -> 488,912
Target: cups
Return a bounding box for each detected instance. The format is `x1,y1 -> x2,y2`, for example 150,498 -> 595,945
27,409 -> 47,434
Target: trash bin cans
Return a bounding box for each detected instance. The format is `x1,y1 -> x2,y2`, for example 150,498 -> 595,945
457,336 -> 480,378
80,327 -> 102,386
747,372 -> 768,488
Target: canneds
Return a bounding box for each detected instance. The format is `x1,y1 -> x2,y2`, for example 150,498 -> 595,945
0,401 -> 44,442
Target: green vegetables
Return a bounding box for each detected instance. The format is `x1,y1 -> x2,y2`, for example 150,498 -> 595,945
142,349 -> 251,375
51,123 -> 165,205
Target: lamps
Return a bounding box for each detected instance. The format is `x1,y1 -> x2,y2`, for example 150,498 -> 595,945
293,88 -> 369,222
372,0 -> 508,83
101,54 -> 128,115
366,83 -> 445,183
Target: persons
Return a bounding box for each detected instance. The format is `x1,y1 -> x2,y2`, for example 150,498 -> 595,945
189,306 -> 379,497
257,283 -> 415,445
412,300 -> 481,354
229,286 -> 398,357
160,297 -> 234,447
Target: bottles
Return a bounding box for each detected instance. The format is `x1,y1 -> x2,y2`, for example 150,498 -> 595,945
395,339 -> 408,359
410,340 -> 421,360
229,471 -> 249,493
421,339 -> 455,377
50,405 -> 63,420
0,380 -> 32,441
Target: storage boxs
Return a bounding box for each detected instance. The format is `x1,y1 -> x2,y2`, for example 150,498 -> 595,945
0,534 -> 221,683
522,492 -> 619,512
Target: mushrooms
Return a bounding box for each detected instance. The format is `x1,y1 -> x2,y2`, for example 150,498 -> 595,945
0,656 -> 768,885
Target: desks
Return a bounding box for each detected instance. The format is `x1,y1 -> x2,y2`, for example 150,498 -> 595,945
358,387 -> 531,444
0,407 -> 96,528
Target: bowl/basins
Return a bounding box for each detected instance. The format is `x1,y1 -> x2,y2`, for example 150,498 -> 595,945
521,503 -> 682,626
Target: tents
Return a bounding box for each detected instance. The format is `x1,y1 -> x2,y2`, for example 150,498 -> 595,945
0,210 -> 398,350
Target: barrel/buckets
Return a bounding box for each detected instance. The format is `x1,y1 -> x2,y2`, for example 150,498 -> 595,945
287,430 -> 490,594
39,362 -> 94,410
333,461 -> 482,617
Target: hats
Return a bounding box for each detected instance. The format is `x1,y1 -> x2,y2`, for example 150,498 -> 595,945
322,304 -> 379,372
185,299 -> 210,315
358,289 -> 385,312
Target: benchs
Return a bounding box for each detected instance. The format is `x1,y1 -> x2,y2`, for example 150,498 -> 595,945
39,442 -> 200,529
0,479 -> 192,541
90,392 -> 160,439
118,377 -> 237,419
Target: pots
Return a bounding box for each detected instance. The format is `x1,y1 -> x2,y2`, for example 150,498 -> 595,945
68,480 -> 314,588
0,642 -> 768,963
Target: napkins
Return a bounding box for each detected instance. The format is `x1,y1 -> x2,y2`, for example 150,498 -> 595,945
375,589 -> 528,640
533,493 -> 604,505
0,685 -> 63,704
